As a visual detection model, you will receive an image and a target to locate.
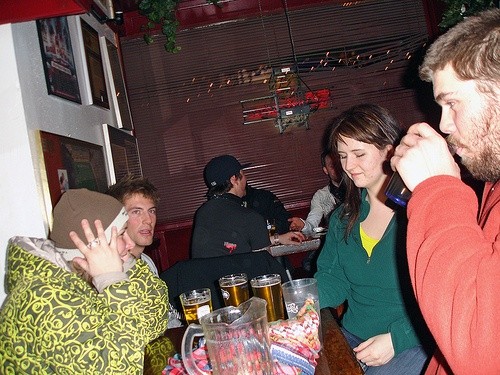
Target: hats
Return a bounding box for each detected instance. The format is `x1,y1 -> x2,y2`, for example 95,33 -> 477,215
203,155 -> 251,189
49,187 -> 129,262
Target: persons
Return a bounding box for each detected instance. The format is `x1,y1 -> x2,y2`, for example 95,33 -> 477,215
288,152 -> 348,237
0,189 -> 176,375
106,172 -> 184,329
190,156 -> 305,259
314,104 -> 436,375
391,5 -> 500,375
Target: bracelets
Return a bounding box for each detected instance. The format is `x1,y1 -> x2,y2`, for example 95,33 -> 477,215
274,234 -> 280,245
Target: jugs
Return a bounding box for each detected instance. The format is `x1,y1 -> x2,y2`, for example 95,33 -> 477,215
180,296 -> 276,375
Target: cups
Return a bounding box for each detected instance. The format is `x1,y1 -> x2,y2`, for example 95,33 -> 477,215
268,225 -> 275,237
250,273 -> 285,322
218,272 -> 250,306
281,277 -> 324,353
383,171 -> 412,208
179,288 -> 214,350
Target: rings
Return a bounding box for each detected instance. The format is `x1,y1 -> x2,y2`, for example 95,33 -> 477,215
88,240 -> 99,248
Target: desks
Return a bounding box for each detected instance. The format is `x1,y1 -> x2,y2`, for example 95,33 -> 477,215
270,225 -> 328,268
143,306 -> 366,375
